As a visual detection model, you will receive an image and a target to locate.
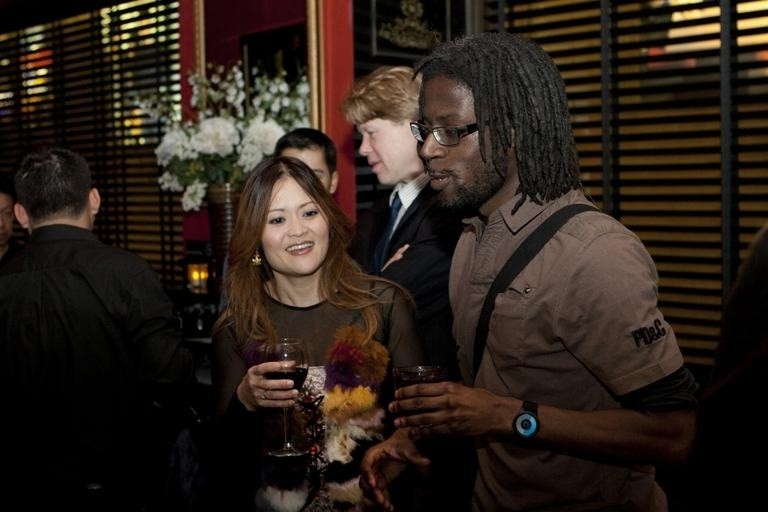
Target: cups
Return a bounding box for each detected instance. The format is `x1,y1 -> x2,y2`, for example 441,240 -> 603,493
390,364 -> 441,418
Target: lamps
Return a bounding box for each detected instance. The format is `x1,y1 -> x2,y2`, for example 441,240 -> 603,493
185,254 -> 211,297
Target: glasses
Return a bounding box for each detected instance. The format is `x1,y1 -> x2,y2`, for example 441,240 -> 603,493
409,118 -> 477,147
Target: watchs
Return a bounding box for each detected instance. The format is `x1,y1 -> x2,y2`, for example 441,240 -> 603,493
512,401 -> 540,440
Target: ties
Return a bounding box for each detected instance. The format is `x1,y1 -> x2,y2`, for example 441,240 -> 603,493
380,192 -> 402,257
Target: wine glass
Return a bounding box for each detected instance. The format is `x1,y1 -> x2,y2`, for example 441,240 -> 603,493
255,339 -> 310,459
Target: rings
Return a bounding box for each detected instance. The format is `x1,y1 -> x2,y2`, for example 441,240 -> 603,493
260,389 -> 267,400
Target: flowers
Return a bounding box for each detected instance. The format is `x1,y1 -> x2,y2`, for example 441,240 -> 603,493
140,60 -> 310,210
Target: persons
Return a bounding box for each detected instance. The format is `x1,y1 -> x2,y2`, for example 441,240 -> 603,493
0,170 -> 23,269
356,28 -> 705,511
1,146 -> 190,512
206,153 -> 429,512
338,63 -> 470,364
216,128 -> 340,314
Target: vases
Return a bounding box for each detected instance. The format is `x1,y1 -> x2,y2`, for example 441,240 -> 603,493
211,181 -> 237,255
